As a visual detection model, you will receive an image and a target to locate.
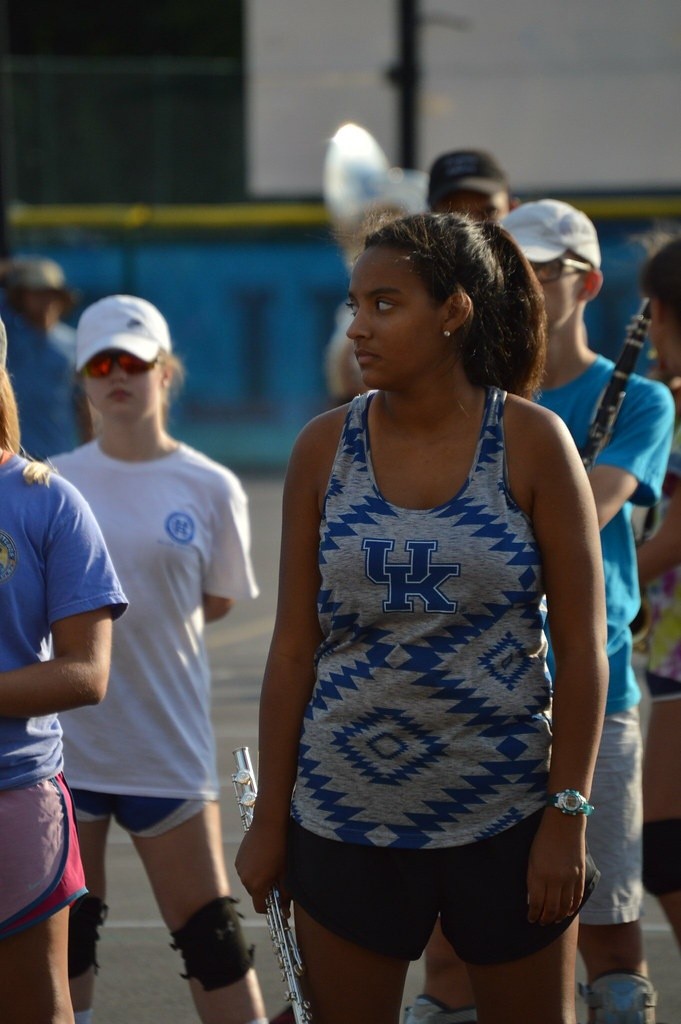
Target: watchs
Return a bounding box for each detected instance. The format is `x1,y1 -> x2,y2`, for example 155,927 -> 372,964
549,789 -> 594,817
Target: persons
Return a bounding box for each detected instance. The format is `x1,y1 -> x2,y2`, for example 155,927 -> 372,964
37,293 -> 264,1024
0,319 -> 133,1024
234,215 -> 610,1024
1,255 -> 87,457
328,148 -> 680,1024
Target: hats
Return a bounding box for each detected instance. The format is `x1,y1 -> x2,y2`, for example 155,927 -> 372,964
501,199 -> 603,269
427,150 -> 508,203
76,296 -> 171,373
14,257 -> 67,293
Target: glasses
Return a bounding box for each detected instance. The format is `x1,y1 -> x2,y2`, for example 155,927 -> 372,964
529,257 -> 591,285
87,353 -> 156,378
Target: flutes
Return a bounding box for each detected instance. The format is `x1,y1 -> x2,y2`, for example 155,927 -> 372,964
577,300 -> 652,466
230,746 -> 317,1024
